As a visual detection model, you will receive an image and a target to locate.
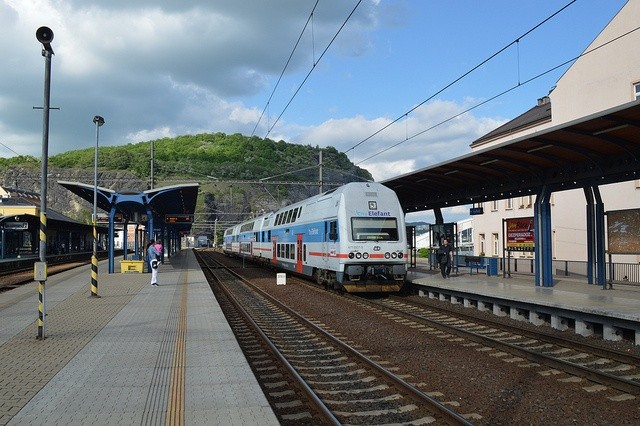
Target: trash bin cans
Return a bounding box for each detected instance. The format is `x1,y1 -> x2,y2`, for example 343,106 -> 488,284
428,249 -> 438,269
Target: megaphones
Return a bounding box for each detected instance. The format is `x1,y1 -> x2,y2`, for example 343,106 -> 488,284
36,26 -> 54,49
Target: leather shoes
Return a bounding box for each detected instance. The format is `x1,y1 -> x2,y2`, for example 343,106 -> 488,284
443,275 -> 446,279
446,273 -> 450,278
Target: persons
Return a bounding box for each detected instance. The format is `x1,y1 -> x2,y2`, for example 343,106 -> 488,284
437,238 -> 453,279
154,240 -> 163,265
146,239 -> 162,287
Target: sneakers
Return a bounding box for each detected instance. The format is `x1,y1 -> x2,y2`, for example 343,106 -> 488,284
152,284 -> 155,287
160,260 -> 161,262
157,261 -> 160,266
154,283 -> 159,287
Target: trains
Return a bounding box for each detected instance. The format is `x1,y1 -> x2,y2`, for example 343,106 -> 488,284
222,181 -> 408,299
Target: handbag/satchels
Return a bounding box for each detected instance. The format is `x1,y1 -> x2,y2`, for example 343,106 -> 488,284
152,261 -> 157,268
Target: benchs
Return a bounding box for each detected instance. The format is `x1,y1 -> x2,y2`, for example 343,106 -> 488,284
459,263 -> 486,275
